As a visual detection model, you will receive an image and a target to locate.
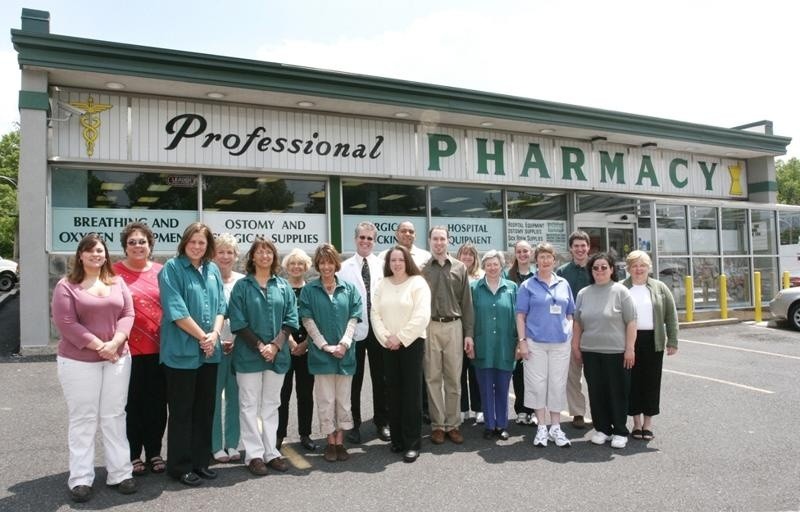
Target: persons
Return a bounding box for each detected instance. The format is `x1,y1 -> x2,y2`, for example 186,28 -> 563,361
378,219 -> 431,272
514,244 -> 576,450
420,226 -> 474,446
109,223 -> 166,473
457,242 -> 486,426
501,240 -> 539,427
156,220 -> 225,487
571,253 -> 637,448
214,233 -> 246,464
555,230 -> 595,429
299,243 -> 362,464
51,234 -> 137,503
334,221 -> 390,443
228,238 -> 299,479
469,250 -> 519,441
276,248 -> 320,452
369,244 -> 431,464
616,250 -> 680,440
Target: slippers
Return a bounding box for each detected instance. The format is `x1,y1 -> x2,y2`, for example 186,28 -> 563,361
213,447 -> 230,463
643,425 -> 653,440
631,429 -> 643,439
132,458 -> 145,474
224,446 -> 241,462
146,456 -> 166,472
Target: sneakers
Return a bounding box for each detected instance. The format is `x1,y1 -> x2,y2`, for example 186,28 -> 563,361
516,413 -> 528,424
476,411 -> 484,423
336,443 -> 349,461
574,416 -> 584,428
325,444 -> 337,461
73,486 -> 91,500
611,434 -> 628,448
533,427 -> 549,446
529,413 -> 539,426
117,478 -> 138,492
591,431 -> 614,445
460,411 -> 470,424
548,427 -> 571,447
269,457 -> 288,471
249,458 -> 269,475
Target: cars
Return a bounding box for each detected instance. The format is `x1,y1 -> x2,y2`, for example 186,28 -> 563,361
768,285 -> 800,330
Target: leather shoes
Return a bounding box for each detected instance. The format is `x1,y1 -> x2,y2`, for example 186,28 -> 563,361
378,425 -> 391,440
391,443 -> 401,452
300,435 -> 314,450
431,429 -> 444,444
345,428 -> 360,442
199,468 -> 218,479
405,450 -> 421,462
180,472 -> 202,487
484,429 -> 495,439
275,437 -> 283,450
496,427 -> 509,440
449,428 -> 463,443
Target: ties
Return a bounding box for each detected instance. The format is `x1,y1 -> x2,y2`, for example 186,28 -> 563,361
362,258 -> 371,322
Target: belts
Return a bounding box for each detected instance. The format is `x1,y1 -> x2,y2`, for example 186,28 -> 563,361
432,316 -> 459,322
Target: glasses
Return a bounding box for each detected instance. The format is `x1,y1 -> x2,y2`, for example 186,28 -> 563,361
360,235 -> 373,241
592,265 -> 607,271
400,230 -> 415,233
126,240 -> 148,245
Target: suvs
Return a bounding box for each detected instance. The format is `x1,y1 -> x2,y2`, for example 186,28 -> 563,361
0,254 -> 18,292
